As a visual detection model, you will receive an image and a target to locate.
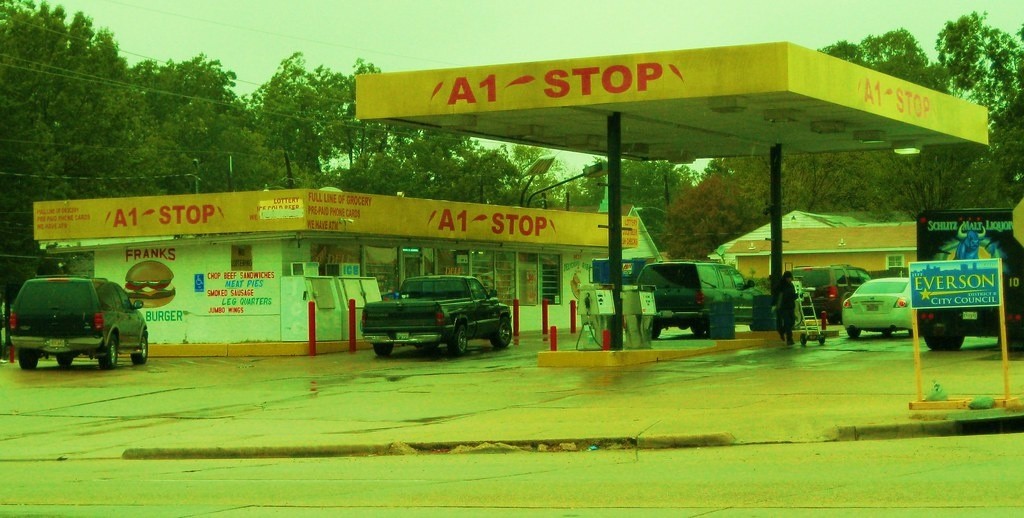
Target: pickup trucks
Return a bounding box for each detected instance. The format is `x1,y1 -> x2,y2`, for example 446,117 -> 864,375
361,273 -> 513,358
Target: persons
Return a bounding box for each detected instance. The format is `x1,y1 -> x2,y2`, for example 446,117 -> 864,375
762,275 -> 772,289
771,271 -> 799,346
740,279 -> 756,290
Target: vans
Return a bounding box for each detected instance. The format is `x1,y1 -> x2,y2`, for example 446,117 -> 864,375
790,265 -> 871,325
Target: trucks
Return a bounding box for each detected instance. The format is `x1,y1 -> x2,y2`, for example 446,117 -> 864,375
914,209 -> 1024,353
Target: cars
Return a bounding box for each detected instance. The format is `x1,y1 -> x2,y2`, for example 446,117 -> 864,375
842,277 -> 917,339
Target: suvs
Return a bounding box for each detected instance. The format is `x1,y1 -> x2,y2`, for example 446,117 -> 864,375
633,258 -> 765,340
10,275 -> 149,370
